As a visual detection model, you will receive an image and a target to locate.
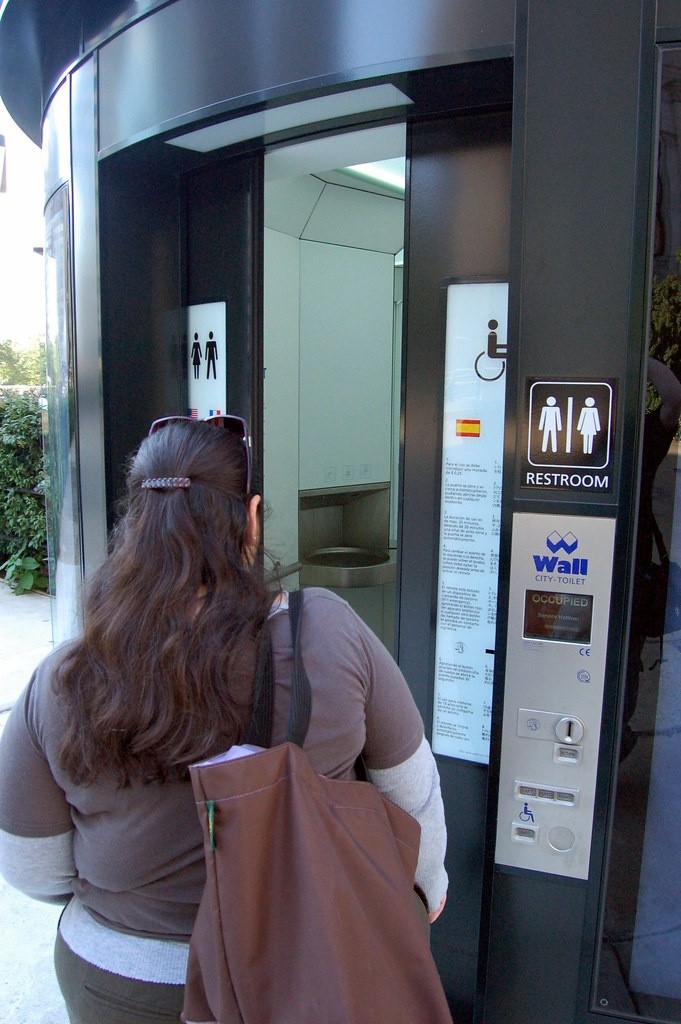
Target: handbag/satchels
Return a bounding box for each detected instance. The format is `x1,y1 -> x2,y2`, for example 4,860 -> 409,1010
178,590 -> 453,1024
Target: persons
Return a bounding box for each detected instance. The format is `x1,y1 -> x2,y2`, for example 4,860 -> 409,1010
0,424 -> 448,1024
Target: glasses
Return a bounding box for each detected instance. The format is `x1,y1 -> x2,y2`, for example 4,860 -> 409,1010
149,415 -> 252,493
